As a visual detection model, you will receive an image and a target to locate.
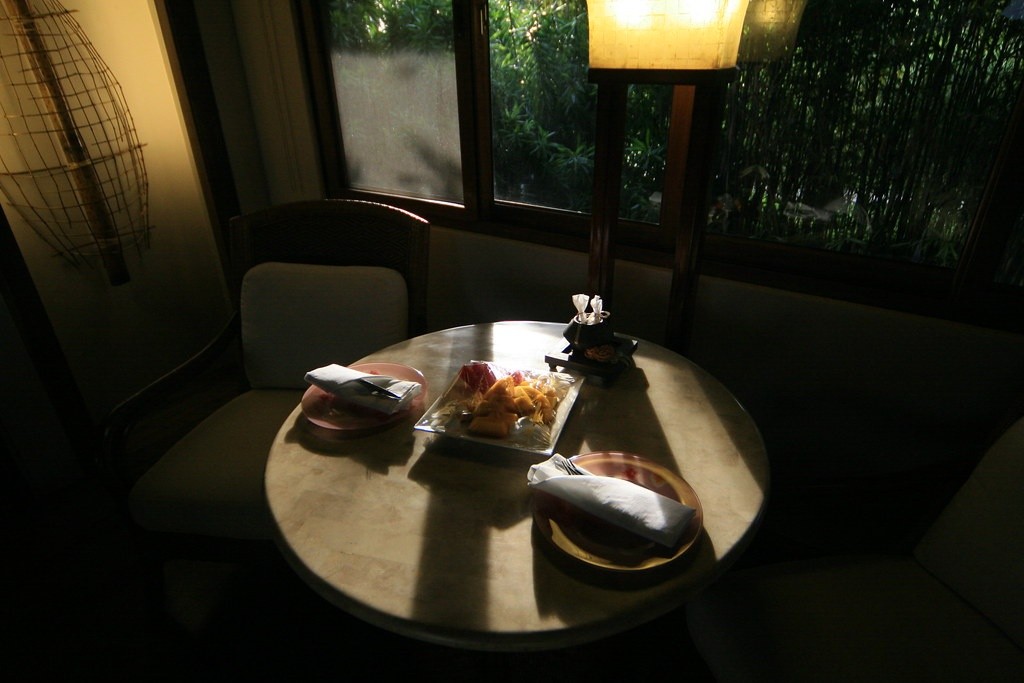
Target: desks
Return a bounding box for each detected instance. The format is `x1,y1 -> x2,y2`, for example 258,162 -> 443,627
265,320 -> 771,683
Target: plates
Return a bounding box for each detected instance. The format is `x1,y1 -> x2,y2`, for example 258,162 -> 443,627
415,360 -> 586,455
531,450 -> 704,571
301,363 -> 426,431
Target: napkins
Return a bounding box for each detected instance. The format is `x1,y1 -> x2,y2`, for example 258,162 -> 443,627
526,453 -> 697,548
302,362 -> 424,416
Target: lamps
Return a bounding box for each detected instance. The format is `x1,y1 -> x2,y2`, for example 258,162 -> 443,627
0,0 -> 156,291
583,0 -> 752,85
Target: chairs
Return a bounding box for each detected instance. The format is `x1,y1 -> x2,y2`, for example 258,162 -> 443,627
642,385 -> 1024,683
93,199 -> 433,683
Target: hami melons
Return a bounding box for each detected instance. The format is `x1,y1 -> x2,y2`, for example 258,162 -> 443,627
456,377 -> 558,436
